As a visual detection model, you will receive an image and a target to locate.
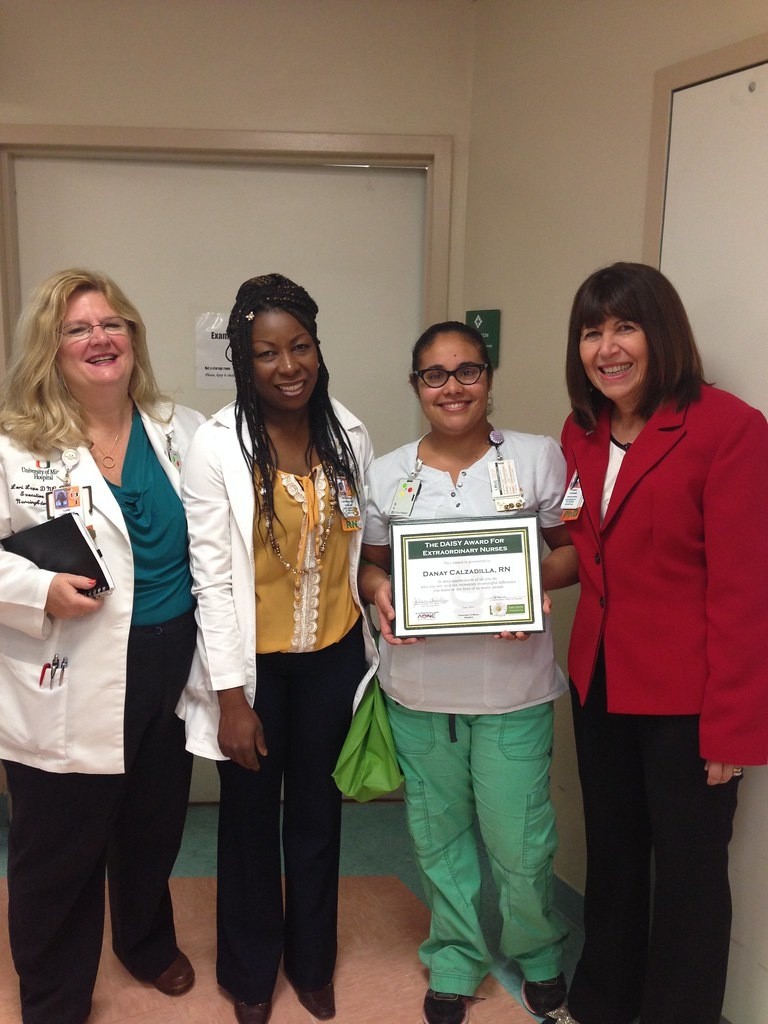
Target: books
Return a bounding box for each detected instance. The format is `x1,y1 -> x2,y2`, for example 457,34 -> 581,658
2,512 -> 114,598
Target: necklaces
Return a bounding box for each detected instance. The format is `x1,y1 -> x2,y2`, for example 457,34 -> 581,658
257,445 -> 337,574
92,406 -> 124,467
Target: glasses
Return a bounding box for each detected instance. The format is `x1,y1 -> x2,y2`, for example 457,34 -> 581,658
62,317 -> 129,339
413,363 -> 489,389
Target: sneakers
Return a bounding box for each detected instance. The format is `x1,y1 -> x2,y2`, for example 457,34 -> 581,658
420,972 -> 567,1024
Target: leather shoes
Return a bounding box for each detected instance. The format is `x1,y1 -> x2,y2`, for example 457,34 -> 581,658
152,951 -> 193,995
236,982 -> 335,1024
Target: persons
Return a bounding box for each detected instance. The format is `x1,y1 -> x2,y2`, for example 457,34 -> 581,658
559,262 -> 768,1022
173,270 -> 373,1024
358,318 -> 570,1023
55,492 -> 66,505
338,483 -> 344,492
2,267 -> 214,1024
